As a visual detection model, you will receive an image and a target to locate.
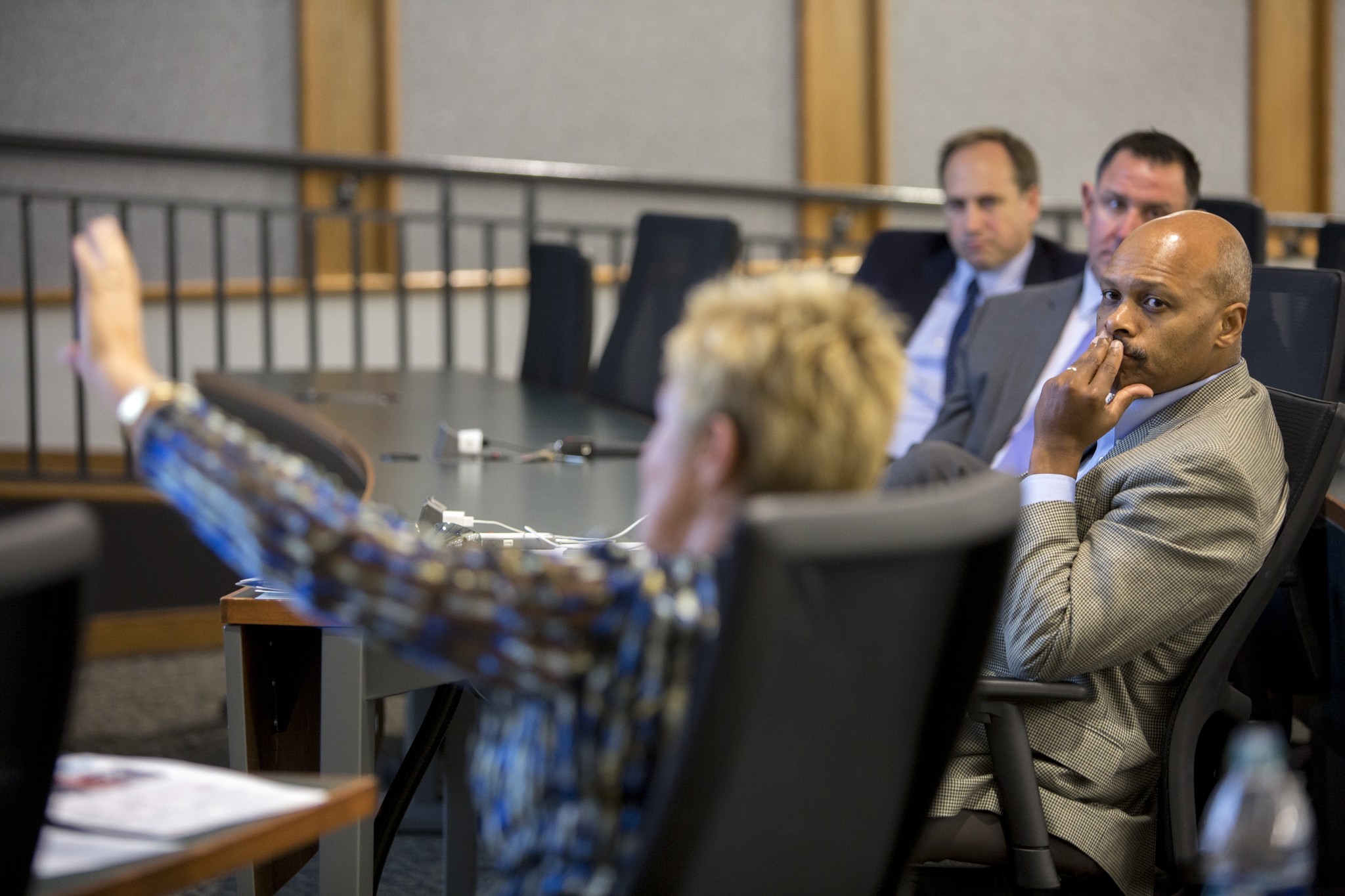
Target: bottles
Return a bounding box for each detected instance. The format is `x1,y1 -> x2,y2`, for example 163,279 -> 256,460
1201,721 -> 1315,896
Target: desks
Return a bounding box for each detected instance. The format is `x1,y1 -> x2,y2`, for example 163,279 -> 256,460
27,742 -> 377,896
195,357 -> 653,894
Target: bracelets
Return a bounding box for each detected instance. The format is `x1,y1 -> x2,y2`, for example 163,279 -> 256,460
113,386 -> 191,436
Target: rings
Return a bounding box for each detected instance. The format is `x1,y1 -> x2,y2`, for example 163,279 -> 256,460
1065,366 -> 1076,371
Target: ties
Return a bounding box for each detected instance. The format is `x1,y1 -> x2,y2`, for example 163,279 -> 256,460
994,324 -> 1096,477
945,279 -> 979,395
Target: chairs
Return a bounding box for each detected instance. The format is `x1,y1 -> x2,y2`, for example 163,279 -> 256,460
593,210 -> 741,423
518,239 -> 594,395
907,188 -> 1345,896
616,466 -> 1020,896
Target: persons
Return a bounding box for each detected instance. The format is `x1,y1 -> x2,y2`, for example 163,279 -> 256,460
904,209 -> 1290,896
63,212 -> 906,894
879,127 -> 1200,493
838,127 -> 1098,486
